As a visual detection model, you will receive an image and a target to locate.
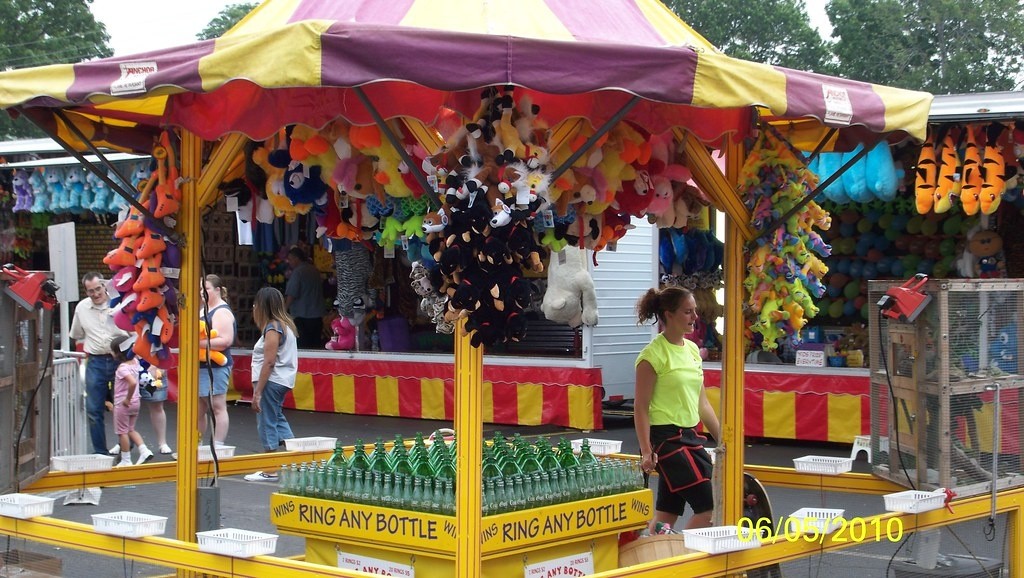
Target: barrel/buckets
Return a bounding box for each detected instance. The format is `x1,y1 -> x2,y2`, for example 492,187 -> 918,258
617,534 -> 697,569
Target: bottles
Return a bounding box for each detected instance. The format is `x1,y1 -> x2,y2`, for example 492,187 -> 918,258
277,431 -> 645,517
707,344 -> 722,361
371,330 -> 379,351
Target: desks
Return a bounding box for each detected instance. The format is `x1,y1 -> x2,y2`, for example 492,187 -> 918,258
700,361 -> 1019,453
168,347 -> 605,432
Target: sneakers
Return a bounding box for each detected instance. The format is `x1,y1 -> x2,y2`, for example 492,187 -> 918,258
109,444 -> 121,455
244,471 -> 279,482
134,450 -> 153,465
159,443 -> 172,453
116,462 -> 132,465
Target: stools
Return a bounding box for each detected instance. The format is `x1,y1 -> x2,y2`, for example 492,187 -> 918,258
851,435 -> 892,463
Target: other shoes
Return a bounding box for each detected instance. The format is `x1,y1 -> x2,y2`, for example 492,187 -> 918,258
172,453 -> 178,459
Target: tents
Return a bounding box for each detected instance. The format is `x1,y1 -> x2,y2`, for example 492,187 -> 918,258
0,0 -> 935,578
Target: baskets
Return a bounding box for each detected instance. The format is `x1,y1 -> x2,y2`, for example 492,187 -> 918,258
283,437 -> 338,452
91,511 -> 168,537
792,455 -> 854,475
571,438 -> 623,456
788,507 -> 845,534
0,493 -> 56,519
704,448 -> 716,465
883,490 -> 947,513
52,454 -> 115,472
198,445 -> 236,458
195,528 -> 279,558
683,526 -> 765,554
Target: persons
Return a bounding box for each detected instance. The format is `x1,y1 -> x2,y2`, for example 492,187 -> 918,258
171,274 -> 238,462
634,284 -> 719,538
67,270 -> 134,455
108,337 -> 154,467
283,247 -> 325,349
244,287 -> 298,480
108,338 -> 172,454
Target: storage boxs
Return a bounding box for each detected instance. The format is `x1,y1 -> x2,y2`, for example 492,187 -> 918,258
195,527 -> 278,558
0,493 -> 54,520
683,526 -> 765,554
196,444 -> 236,462
891,554 -> 1005,578
704,448 -> 716,464
788,508 -> 846,534
792,455 -> 854,475
283,435 -> 337,451
51,453 -> 114,474
570,438 -> 624,457
90,510 -> 168,538
882,488 -> 947,513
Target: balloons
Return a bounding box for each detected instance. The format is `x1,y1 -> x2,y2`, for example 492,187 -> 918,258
817,206 -> 978,321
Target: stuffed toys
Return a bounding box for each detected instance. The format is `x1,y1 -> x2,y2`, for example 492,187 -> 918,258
912,123 -> 1024,278
10,85 -> 869,399
